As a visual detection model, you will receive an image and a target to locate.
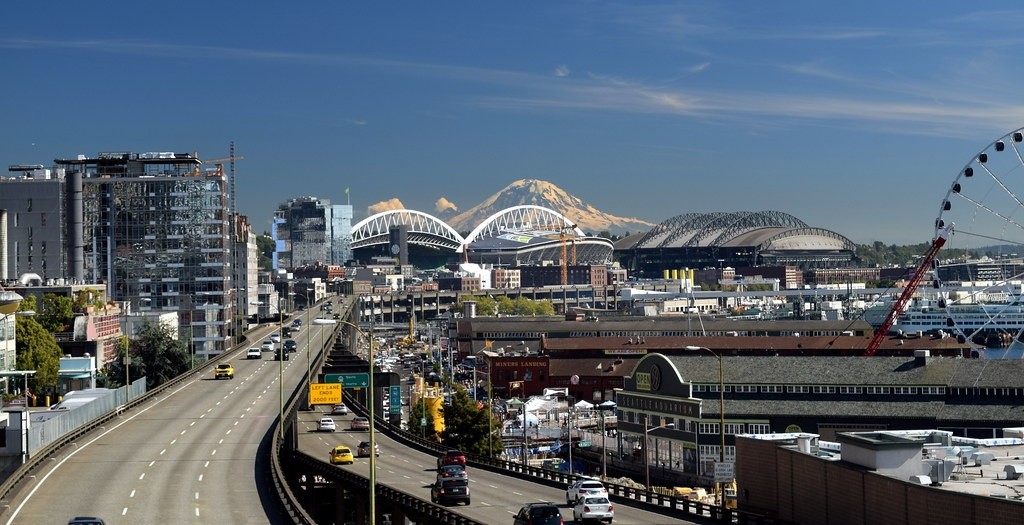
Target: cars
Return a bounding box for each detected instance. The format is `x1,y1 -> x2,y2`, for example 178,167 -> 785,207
357,331 -> 451,432
331,403 -> 347,415
513,500 -> 565,525
292,319 -> 303,326
436,450 -> 467,470
282,340 -> 298,352
268,334 -> 281,343
279,327 -> 293,339
571,494 -> 615,525
431,478 -> 471,506
316,417 -> 336,431
564,480 -> 609,506
289,324 -> 302,333
67,515 -> 105,525
247,348 -> 263,359
316,294 -> 348,319
260,339 -> 275,351
436,464 -> 469,485
357,441 -> 380,458
273,347 -> 289,361
329,445 -> 354,464
350,417 -> 370,431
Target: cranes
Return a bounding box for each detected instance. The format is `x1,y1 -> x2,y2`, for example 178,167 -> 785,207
173,155 -> 245,171
862,222 -> 955,356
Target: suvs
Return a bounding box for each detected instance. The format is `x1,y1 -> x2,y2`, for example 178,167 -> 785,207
215,363 -> 234,380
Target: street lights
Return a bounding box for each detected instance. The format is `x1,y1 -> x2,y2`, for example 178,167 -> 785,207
287,292 -> 313,412
313,319 -> 376,525
306,288 -> 325,367
685,344 -> 727,525
250,301 -> 285,439
125,297 -> 153,402
644,417 -> 675,492
466,355 -> 493,459
